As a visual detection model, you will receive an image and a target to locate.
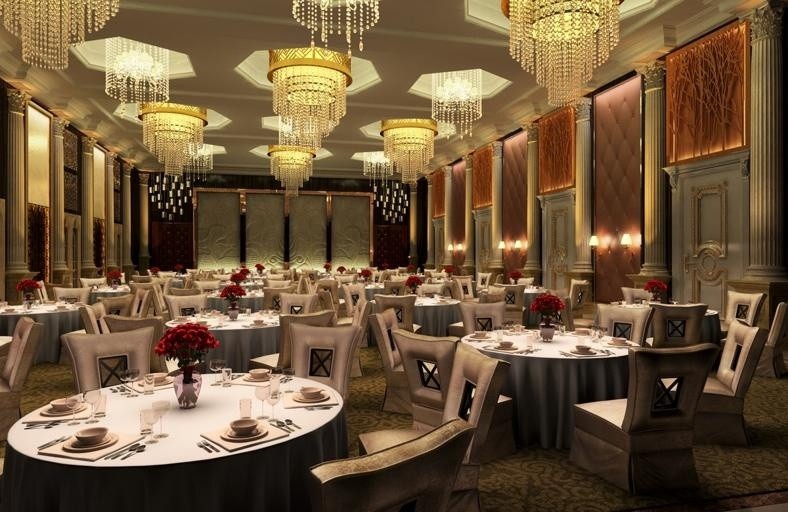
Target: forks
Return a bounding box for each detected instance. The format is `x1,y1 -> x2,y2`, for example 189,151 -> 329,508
196,440 -> 221,454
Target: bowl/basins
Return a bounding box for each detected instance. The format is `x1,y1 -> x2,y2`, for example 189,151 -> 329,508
73,428 -> 108,445
249,369 -> 269,378
51,398 -> 76,411
299,387 -> 320,399
150,372 -> 168,382
229,419 -> 258,436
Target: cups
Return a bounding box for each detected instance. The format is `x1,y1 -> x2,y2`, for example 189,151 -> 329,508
143,374 -> 155,395
238,398 -> 252,419
139,410 -> 152,434
221,368 -> 232,388
93,395 -> 106,417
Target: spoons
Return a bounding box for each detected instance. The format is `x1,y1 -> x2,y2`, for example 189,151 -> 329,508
108,443 -> 145,461
276,419 -> 301,432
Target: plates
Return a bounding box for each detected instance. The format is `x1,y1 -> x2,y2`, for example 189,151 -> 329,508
292,394 -> 330,403
139,380 -> 174,388
242,376 -> 268,382
219,428 -> 269,442
462,329 -> 631,358
42,406 -> 86,416
62,433 -> 120,452
166,312 -> 279,328
0,304 -> 71,316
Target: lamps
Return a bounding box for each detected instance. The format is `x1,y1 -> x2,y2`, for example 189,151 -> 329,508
355,117 -> 464,189
448,232 -> 641,257
0,0 -> 123,73
346,149 -> 399,193
247,142 -> 337,201
64,33 -> 200,107
405,67 -> 523,143
498,1 -> 621,110
110,99 -> 231,181
173,139 -> 229,185
232,46 -> 388,154
287,1 -> 383,61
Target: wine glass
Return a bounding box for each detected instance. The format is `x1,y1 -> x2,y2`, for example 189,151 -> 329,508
64,395 -> 81,425
254,386 -> 268,418
83,389 -> 101,425
119,368 -> 139,398
208,359 -> 226,386
267,391 -> 280,422
282,368 -> 294,393
152,400 -> 171,439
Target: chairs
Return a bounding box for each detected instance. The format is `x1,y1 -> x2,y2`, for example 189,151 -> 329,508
0,262 -> 788,512
